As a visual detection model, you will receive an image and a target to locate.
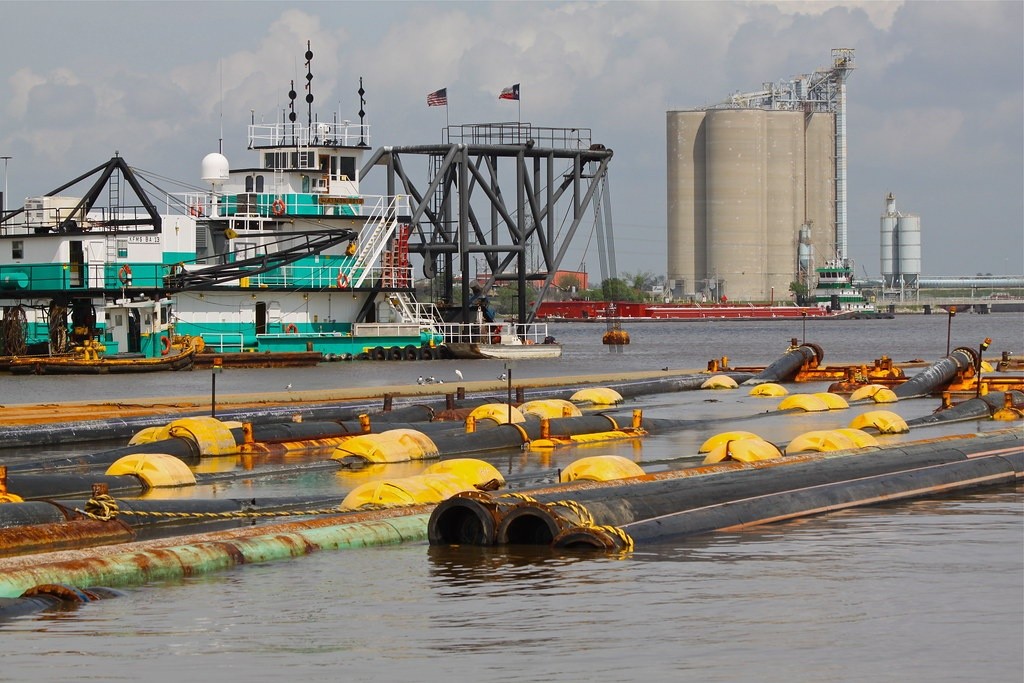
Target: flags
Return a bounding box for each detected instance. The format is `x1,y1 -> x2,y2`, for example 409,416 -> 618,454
499,84 -> 519,101
427,88 -> 446,106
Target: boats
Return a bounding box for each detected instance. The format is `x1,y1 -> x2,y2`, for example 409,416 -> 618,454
192,349 -> 322,367
810,258 -> 872,313
0,40 -> 564,369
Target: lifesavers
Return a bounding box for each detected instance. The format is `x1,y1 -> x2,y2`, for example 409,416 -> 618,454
336,273 -> 350,289
286,324 -> 298,334
119,265 -> 132,285
161,336 -> 171,355
191,203 -> 203,216
272,200 -> 286,216
